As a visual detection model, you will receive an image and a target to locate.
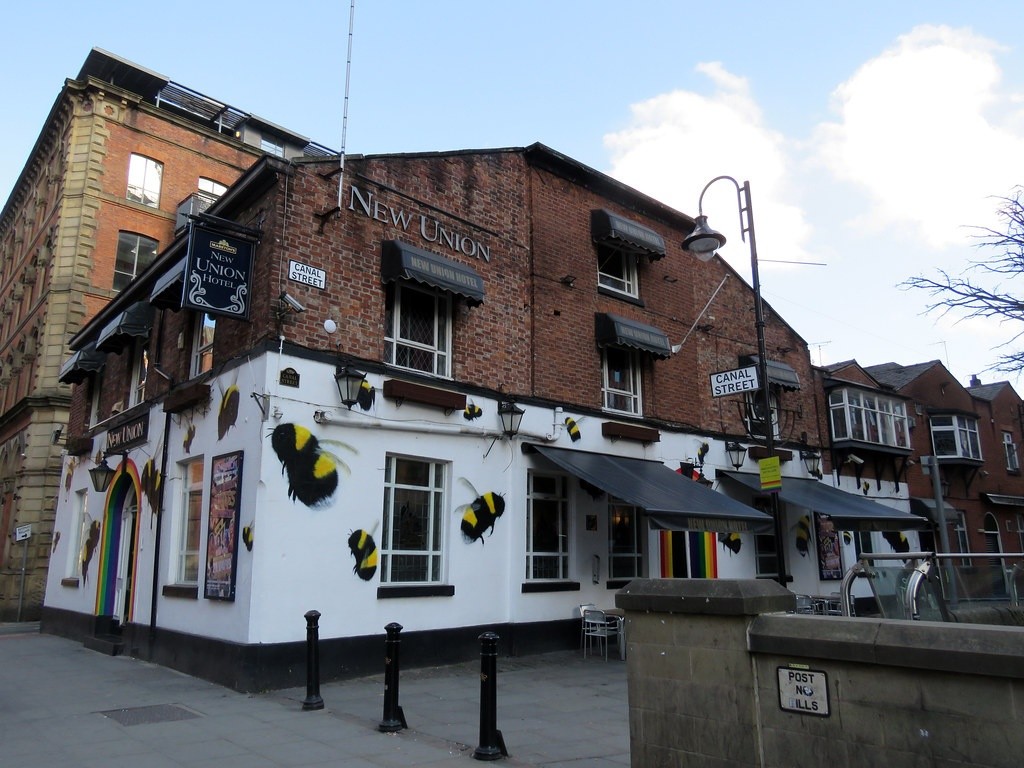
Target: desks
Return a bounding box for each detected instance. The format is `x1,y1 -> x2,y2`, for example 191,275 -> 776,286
602,609 -> 626,661
813,595 -> 841,616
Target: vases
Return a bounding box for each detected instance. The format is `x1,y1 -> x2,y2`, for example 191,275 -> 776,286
384,379 -> 466,411
749,447 -> 792,460
68,438 -> 94,456
603,422 -> 660,443
162,384 -> 210,413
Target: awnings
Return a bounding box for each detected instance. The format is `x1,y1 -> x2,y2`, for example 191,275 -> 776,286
716,469 -> 929,531
590,208 -> 666,264
381,240 -> 486,312
57,341 -> 106,386
979,492 -> 1024,507
95,299 -> 156,357
520,442 -> 775,536
147,255 -> 186,313
594,312 -> 672,363
738,356 -> 802,394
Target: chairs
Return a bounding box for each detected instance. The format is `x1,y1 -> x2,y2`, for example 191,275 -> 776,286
797,592 -> 856,616
579,605 -> 625,660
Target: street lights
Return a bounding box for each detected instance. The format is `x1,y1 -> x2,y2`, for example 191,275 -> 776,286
679,176 -> 787,589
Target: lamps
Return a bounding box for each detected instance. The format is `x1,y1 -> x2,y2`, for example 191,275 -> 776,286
726,439 -> 748,471
693,464 -> 714,489
906,460 -> 915,467
800,448 -> 821,477
696,324 -> 716,331
497,383 -> 526,440
980,471 -> 989,478
560,275 -> 577,285
776,347 -> 794,353
87,449 -> 128,492
333,345 -> 367,412
